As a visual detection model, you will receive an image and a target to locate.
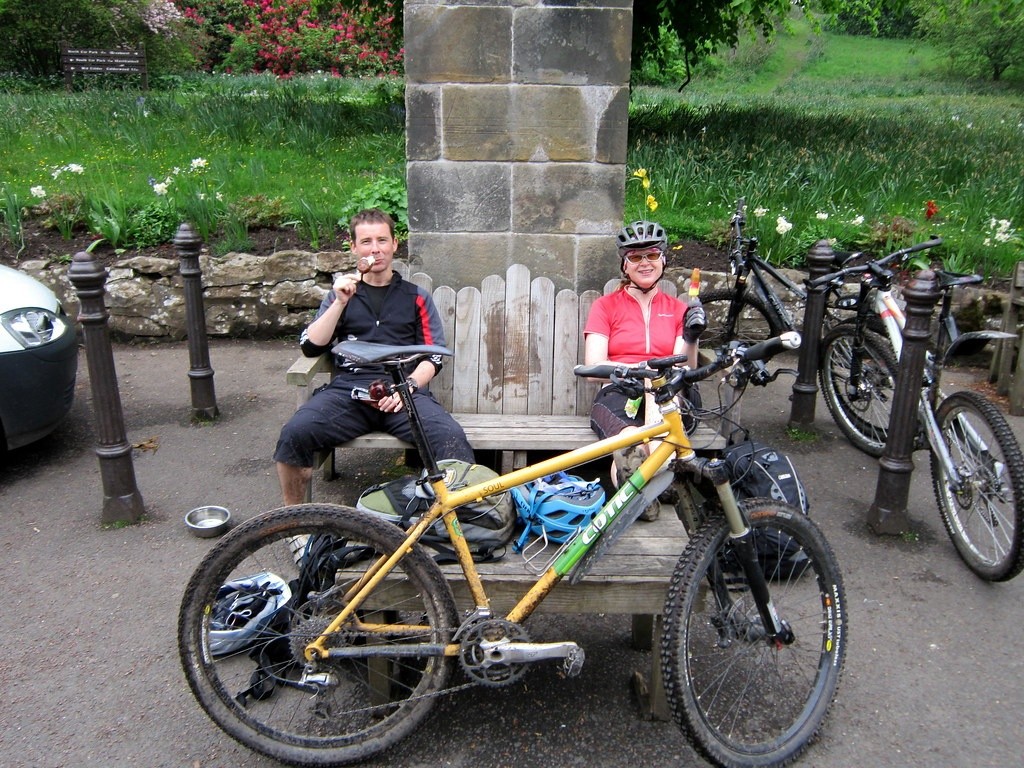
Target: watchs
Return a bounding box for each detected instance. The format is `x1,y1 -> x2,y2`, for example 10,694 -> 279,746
405,377 -> 418,393
638,360 -> 647,369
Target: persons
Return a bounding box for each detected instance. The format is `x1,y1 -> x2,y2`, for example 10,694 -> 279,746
272,210 -> 475,567
584,221 -> 708,521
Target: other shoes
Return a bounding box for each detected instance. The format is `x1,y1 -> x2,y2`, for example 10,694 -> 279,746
283,533 -> 316,568
614,445 -> 660,522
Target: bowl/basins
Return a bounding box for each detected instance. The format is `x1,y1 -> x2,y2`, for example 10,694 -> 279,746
185,506 -> 230,536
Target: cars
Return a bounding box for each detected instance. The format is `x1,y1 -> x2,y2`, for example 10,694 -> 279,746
0,262 -> 78,454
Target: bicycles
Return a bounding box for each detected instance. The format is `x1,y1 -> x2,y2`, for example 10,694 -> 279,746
176,340 -> 848,768
699,198 -> 924,442
809,235 -> 1024,584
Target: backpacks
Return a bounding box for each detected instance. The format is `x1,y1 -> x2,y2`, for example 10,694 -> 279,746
719,440 -> 814,577
356,458 -> 517,566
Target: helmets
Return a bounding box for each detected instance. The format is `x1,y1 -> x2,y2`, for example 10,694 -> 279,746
205,571 -> 293,657
507,470 -> 606,554
615,220 -> 668,251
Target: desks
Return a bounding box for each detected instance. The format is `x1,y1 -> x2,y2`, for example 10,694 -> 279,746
334,507 -> 712,722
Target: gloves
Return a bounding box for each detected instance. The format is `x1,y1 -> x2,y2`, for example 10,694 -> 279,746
682,305 -> 708,343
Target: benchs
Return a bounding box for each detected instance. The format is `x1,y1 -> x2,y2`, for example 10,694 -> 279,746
287,261 -> 745,488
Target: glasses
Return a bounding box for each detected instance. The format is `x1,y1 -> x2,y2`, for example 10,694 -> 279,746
623,250 -> 665,264
